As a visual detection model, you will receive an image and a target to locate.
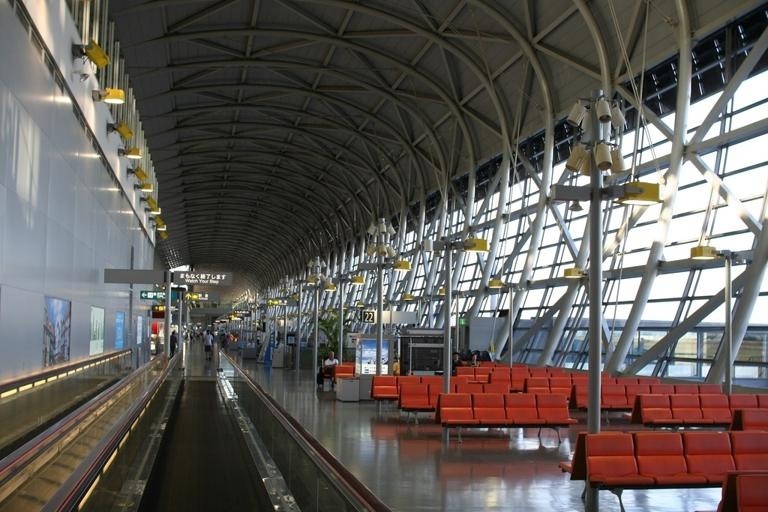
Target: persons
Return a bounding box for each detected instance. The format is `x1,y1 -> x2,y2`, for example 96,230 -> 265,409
324,351 -> 339,391
169,329 -> 233,361
452,351 -> 481,376
393,351 -> 401,376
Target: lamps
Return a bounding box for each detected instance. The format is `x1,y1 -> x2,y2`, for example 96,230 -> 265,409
73,40 -> 169,241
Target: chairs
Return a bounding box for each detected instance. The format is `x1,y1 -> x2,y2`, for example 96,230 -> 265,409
369,360 -> 767,448
334,362 -> 355,385
556,432 -> 767,512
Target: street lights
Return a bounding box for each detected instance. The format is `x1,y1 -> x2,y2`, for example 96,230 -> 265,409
690,247 -> 733,394
307,256 -> 337,372
273,274 -> 300,368
338,276 -> 365,365
566,88 -> 661,431
425,237 -> 491,395
369,216 -> 412,375
490,280 -> 513,368
